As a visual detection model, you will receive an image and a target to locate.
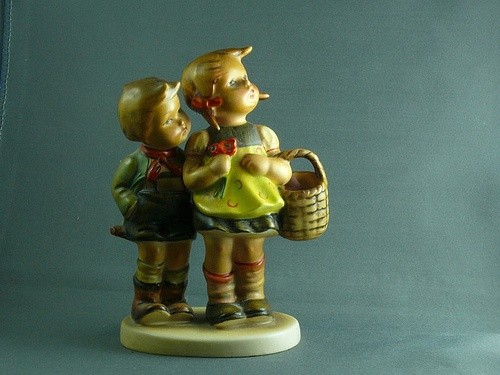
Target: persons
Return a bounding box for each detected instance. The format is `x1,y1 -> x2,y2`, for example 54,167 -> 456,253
182,46 -> 292,327
109,77 -> 196,326
